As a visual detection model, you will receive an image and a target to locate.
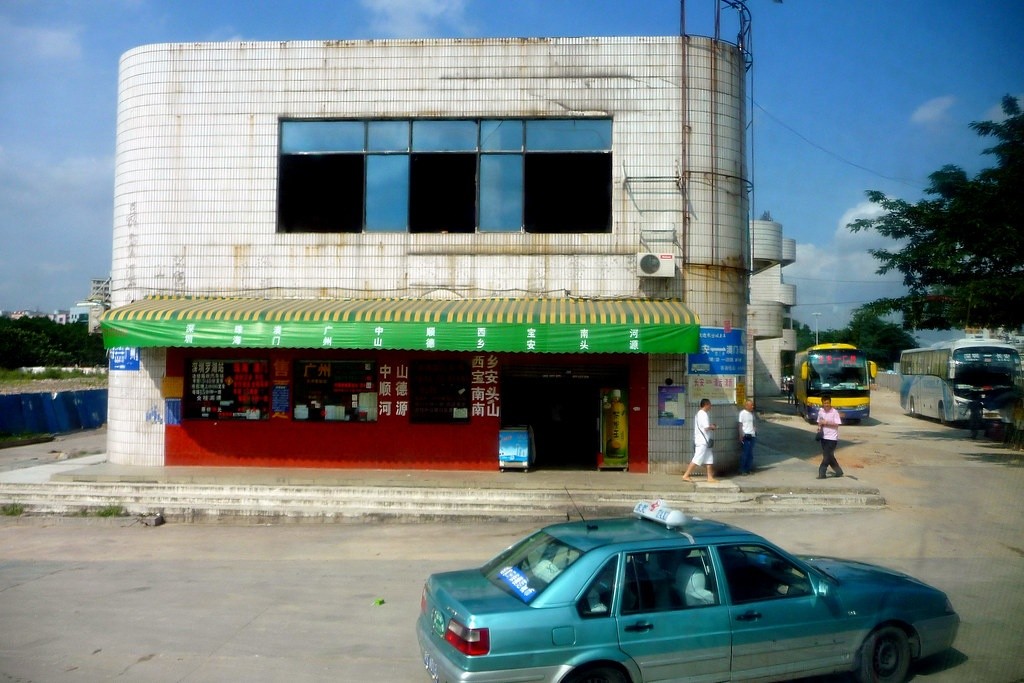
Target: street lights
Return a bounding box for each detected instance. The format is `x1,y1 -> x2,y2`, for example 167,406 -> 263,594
811,311 -> 823,346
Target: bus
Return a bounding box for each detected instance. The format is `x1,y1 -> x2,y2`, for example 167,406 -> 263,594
899,338 -> 1024,426
793,343 -> 877,425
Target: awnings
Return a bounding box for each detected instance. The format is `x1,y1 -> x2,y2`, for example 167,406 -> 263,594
100,294 -> 700,355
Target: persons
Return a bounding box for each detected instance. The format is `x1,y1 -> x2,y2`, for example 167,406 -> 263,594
681,398 -> 720,483
815,395 -> 844,480
738,399 -> 758,476
963,395 -> 983,439
781,378 -> 794,405
847,373 -> 863,385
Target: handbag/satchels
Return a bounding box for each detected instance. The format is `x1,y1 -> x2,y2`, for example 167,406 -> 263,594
707,438 -> 714,448
815,426 -> 823,441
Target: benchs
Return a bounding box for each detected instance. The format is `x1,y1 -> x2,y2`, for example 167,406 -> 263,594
536,561 -> 607,613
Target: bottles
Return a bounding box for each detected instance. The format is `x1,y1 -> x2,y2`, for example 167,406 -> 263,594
606,390 -> 626,458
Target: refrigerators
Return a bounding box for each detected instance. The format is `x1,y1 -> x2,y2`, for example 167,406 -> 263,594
498,427 -> 529,468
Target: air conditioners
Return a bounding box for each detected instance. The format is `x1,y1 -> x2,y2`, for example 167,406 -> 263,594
89,305 -> 105,333
637,252 -> 676,278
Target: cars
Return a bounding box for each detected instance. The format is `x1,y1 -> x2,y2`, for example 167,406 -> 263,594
415,498 -> 963,683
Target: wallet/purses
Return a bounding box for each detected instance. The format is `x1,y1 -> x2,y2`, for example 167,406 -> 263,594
745,434 -> 752,438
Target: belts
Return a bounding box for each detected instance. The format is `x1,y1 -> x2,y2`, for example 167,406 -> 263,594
743,437 -> 755,438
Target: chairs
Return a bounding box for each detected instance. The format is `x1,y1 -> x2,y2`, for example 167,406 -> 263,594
643,552 -> 715,606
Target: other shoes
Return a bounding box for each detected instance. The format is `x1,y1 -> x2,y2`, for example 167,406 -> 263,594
707,479 -> 720,482
836,470 -> 843,476
681,478 -> 694,483
737,471 -> 747,475
746,471 -> 754,474
819,468 -> 826,477
